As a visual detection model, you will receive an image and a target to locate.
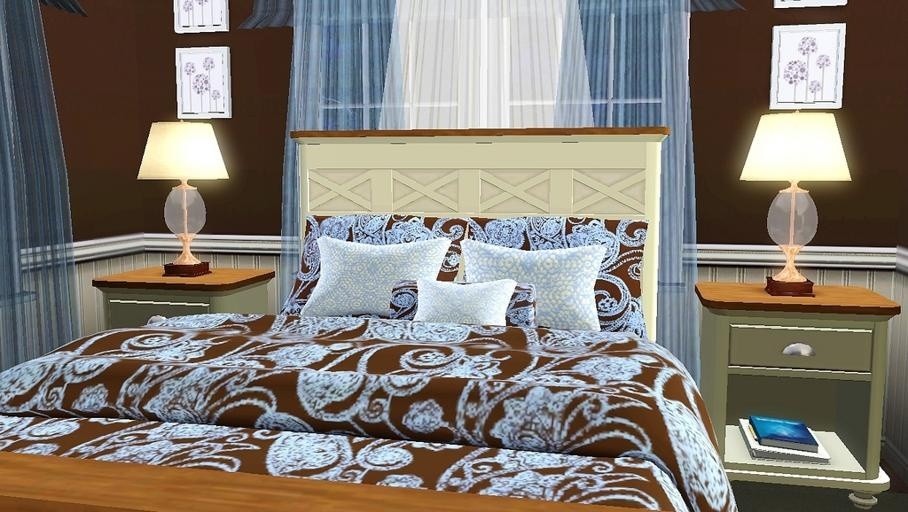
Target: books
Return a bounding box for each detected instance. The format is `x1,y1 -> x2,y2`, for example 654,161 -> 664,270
738,419 -> 831,465
749,417 -> 819,453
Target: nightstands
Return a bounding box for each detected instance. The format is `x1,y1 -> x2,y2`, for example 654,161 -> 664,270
87,260 -> 277,334
686,274 -> 902,511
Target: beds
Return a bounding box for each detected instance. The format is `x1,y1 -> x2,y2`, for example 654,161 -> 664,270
0,124 -> 743,510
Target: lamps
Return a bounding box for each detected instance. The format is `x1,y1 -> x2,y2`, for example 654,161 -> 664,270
134,118 -> 233,278
737,107 -> 856,299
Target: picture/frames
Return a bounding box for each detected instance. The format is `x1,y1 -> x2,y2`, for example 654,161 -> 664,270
768,19 -> 847,111
172,0 -> 231,34
173,46 -> 233,121
772,0 -> 850,10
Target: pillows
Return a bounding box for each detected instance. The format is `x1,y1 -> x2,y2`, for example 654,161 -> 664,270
458,238 -> 607,334
298,235 -> 453,320
280,212 -> 467,322
389,278 -> 538,329
466,215 -> 650,339
413,279 -> 518,328
